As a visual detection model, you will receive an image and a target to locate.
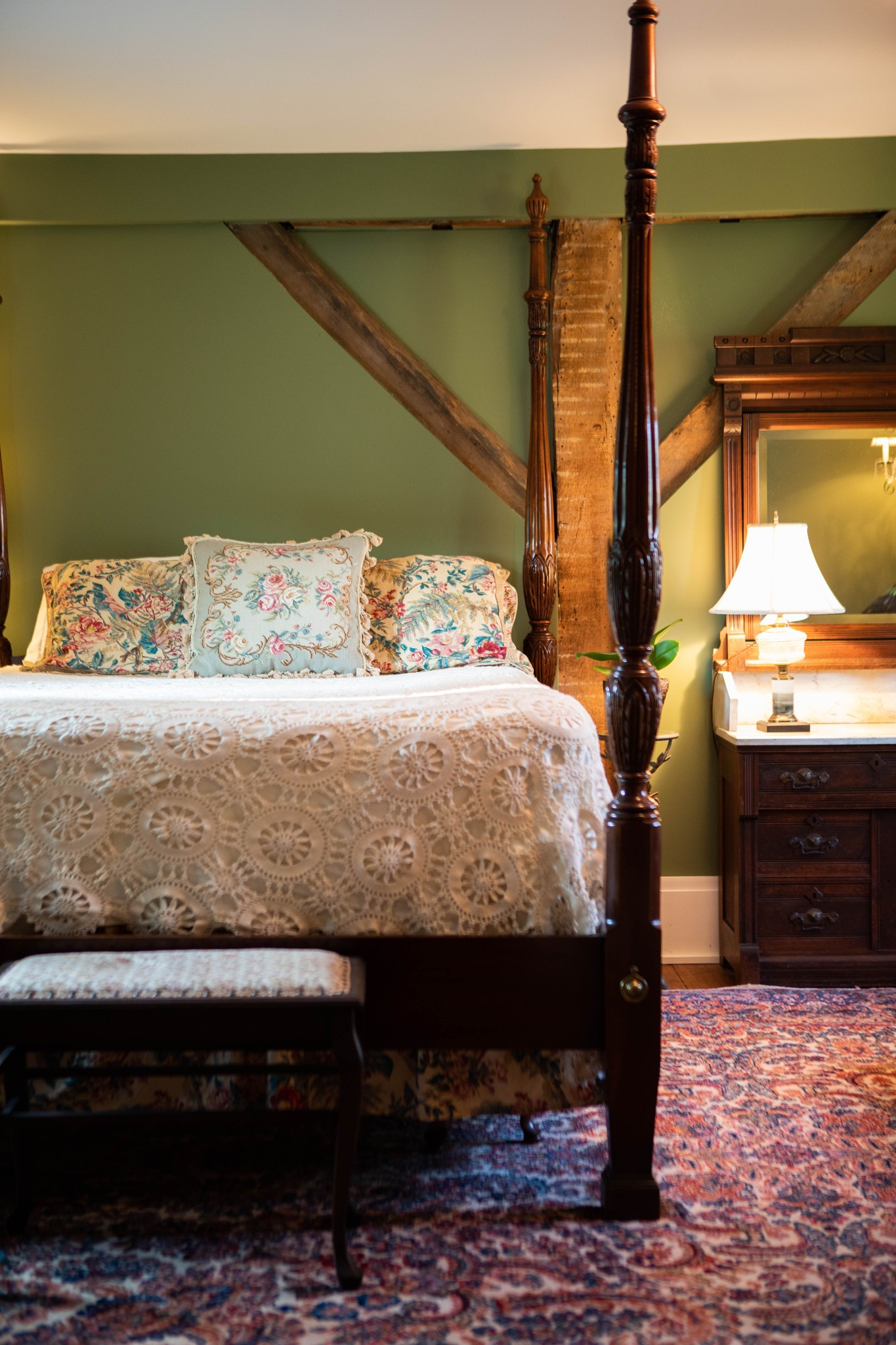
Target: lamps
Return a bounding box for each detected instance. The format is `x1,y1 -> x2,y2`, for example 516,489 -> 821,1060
707,523 -> 845,733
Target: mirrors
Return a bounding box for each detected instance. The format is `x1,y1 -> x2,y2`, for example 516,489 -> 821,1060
743,411 -> 896,641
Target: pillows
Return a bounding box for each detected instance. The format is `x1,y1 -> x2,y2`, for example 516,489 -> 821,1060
187,534 -> 383,680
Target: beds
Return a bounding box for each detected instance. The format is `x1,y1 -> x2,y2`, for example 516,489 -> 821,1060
0,2 -> 666,1220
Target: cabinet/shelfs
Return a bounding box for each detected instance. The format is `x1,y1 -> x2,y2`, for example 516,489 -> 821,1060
735,746 -> 896,991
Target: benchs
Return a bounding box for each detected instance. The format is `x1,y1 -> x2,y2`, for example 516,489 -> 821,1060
0,948 -> 366,1291
